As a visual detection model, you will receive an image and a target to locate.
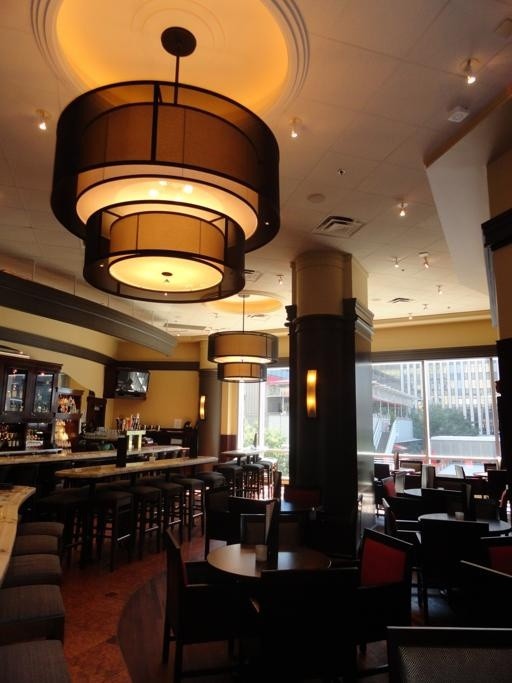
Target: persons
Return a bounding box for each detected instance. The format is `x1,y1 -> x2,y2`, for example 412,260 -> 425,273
120,378 -> 133,390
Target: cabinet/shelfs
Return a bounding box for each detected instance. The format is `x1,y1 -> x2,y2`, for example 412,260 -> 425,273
0,358 -> 56,449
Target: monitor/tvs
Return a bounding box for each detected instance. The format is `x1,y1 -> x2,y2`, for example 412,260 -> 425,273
104,365 -> 150,401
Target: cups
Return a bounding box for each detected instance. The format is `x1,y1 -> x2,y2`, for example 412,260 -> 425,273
253,544 -> 268,562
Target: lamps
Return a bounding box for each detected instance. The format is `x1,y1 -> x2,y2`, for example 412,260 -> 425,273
50,27 -> 282,304
207,292 -> 279,383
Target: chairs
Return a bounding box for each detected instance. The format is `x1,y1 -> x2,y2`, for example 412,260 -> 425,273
374,454 -> 511,625
386,626 -> 510,681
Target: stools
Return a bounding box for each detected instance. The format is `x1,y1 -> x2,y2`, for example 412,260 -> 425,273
1,521 -> 65,682
22,456 -> 279,573
